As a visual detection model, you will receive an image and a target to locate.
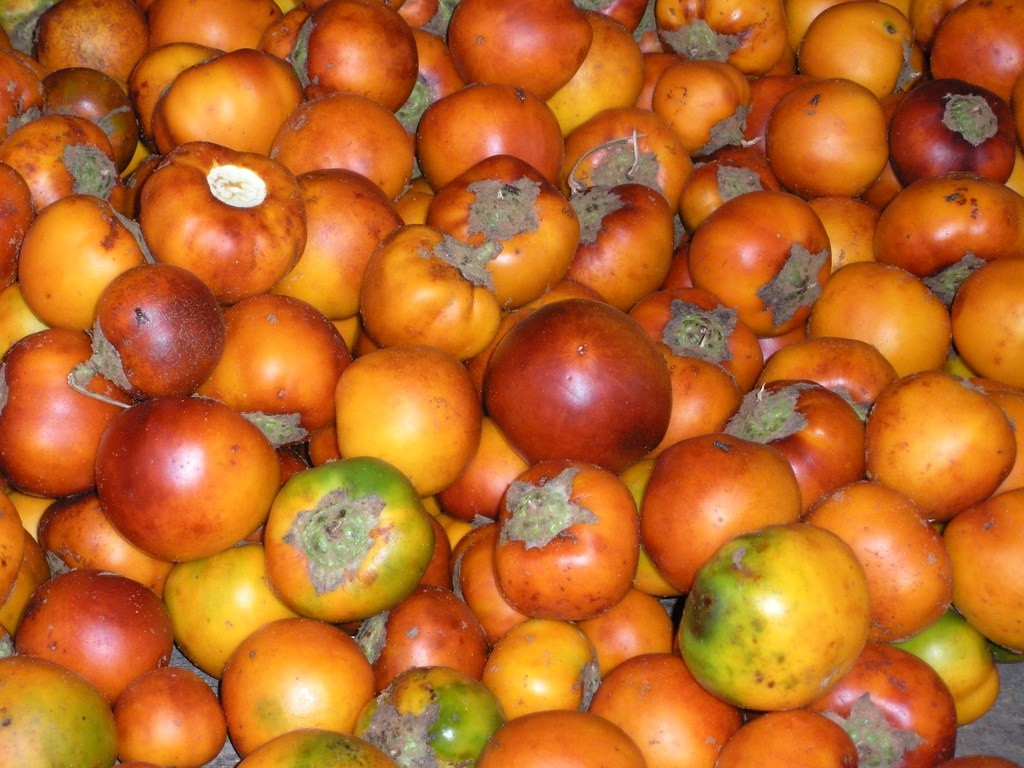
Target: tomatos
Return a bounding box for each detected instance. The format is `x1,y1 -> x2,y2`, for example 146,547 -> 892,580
0,0 -> 1024,767
